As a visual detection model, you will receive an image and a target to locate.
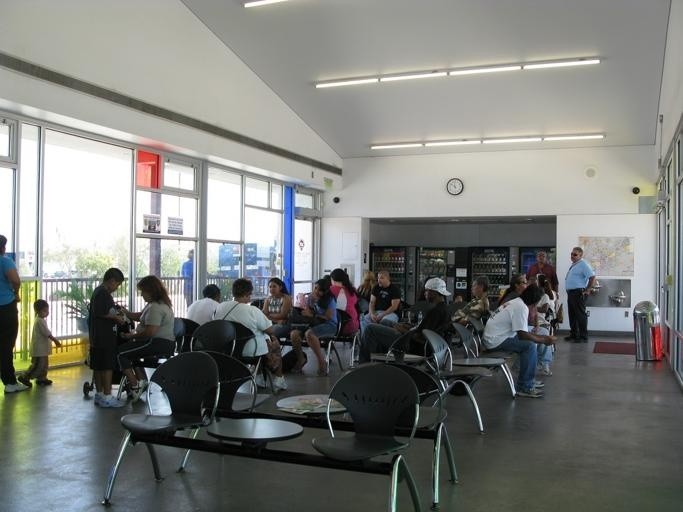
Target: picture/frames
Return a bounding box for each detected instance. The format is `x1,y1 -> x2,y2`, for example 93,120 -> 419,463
520,246 -> 556,284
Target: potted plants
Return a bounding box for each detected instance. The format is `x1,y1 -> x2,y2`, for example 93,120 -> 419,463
54,273 -> 130,332
209,277 -> 234,303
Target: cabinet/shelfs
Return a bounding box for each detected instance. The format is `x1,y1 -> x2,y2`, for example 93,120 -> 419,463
469,247 -> 520,301
417,247 -> 469,304
369,246 -> 415,307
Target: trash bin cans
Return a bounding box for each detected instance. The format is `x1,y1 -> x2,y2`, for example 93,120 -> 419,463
633,301 -> 661,361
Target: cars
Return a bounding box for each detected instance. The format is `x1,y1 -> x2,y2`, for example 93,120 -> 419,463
37,265 -> 68,279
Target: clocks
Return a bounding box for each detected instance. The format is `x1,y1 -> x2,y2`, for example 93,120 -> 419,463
446,177 -> 463,195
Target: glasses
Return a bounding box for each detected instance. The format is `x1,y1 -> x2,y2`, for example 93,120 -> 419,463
571,252 -> 580,257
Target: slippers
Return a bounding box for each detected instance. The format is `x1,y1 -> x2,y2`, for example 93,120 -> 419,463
318,362 -> 328,377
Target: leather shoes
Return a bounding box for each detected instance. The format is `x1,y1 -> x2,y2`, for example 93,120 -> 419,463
562,335 -> 590,344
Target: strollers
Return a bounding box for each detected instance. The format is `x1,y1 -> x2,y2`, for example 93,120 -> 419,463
78,306 -> 145,396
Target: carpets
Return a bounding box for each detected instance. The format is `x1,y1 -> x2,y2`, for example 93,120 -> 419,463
593,341 -> 637,356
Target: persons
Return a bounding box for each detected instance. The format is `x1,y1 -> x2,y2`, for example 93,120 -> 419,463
182,249 -> 193,308
17,299 -> 61,386
353,268 -> 564,398
528,250 -> 560,297
87,267 -> 360,407
0,234 -> 30,393
564,247 -> 596,343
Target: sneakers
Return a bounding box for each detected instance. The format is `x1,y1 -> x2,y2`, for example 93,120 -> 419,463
272,375 -> 289,390
94,392 -> 103,403
4,382 -> 30,393
36,380 -> 53,385
17,375 -> 32,387
516,387 -> 545,399
100,394 -> 124,409
130,379 -> 148,403
536,362 -> 553,378
535,380 -> 545,388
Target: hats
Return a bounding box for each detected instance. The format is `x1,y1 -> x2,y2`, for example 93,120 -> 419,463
423,277 -> 452,297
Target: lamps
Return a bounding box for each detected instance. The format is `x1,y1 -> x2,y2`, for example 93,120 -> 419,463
240,0 -> 297,11
367,133 -> 606,151
316,56 -> 602,90
